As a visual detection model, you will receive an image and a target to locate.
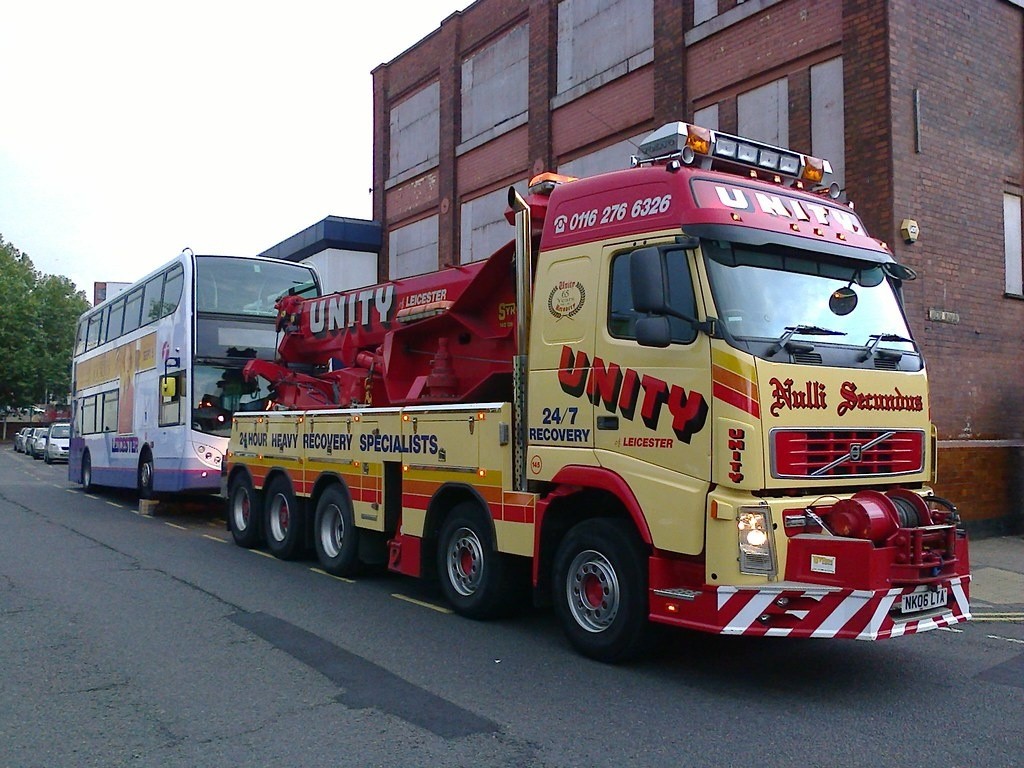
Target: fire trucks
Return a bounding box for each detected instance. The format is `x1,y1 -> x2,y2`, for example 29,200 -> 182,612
218,122 -> 973,672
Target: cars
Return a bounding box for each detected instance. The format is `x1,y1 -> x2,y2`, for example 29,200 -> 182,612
15,422 -> 73,464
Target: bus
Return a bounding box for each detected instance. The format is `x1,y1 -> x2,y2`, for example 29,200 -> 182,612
66,247 -> 323,512
